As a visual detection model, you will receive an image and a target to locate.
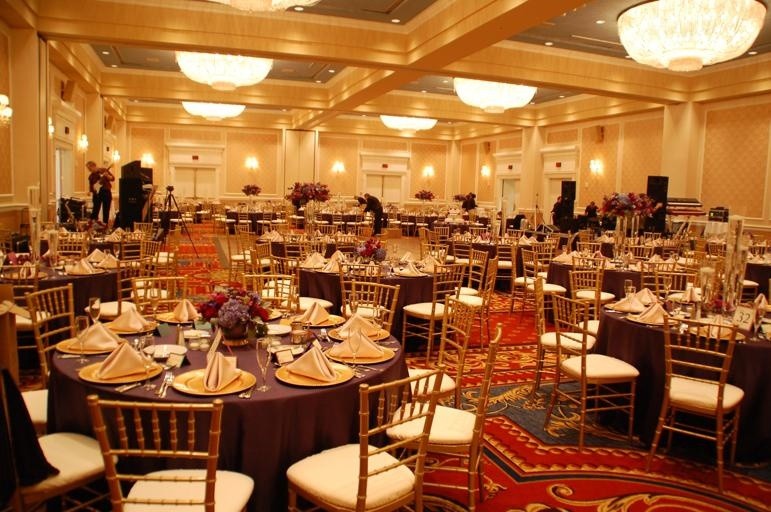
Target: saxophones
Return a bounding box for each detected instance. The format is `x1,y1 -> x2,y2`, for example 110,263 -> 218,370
64,201 -> 84,231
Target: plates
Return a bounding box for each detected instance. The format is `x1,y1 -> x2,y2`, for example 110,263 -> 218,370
255,323 -> 294,335
604,290 -> 771,342
102,321 -> 158,336
289,314 -> 347,328
295,261 -> 450,278
56,336 -> 128,356
182,329 -> 210,340
140,343 -> 188,359
550,256 -> 689,272
0,255 -> 126,280
78,358 -> 162,385
324,345 -> 396,365
274,363 -> 355,388
328,327 -> 391,342
171,368 -> 258,396
156,311 -> 202,324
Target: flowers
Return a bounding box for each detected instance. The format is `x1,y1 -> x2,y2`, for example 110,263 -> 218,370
242,185 -> 260,196
284,181 -> 332,204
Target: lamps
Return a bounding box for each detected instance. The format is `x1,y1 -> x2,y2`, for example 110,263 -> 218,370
380,116 -> 438,135
0,94 -> 12,128
616,0 -> 769,73
207,0 -> 321,12
181,101 -> 246,123
176,51 -> 274,92
454,78 -> 538,114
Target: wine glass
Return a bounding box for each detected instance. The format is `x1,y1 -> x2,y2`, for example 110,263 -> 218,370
347,327 -> 366,380
257,338 -> 274,391
139,336 -> 156,387
74,292 -> 361,389
372,308 -> 384,344
73,316 -> 89,364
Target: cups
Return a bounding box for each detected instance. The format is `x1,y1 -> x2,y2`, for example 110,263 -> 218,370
88,297 -> 101,323
291,330 -> 312,359
200,336 -> 211,352
189,334 -> 200,352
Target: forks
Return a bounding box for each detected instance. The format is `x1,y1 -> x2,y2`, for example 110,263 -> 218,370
153,371 -> 172,394
160,375 -> 175,397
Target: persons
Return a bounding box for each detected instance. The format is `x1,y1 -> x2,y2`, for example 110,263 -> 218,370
552,196 -> 562,226
85,161 -> 114,223
462,192 -> 476,210
584,202 -> 601,238
354,196 -> 367,205
364,192 -> 383,235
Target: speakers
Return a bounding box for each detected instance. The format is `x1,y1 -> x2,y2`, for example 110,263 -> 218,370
141,166 -> 154,185
643,175 -> 668,237
481,141 -> 491,154
105,115 -> 115,130
62,80 -> 78,102
119,177 -> 142,230
558,180 -> 576,219
588,126 -> 603,141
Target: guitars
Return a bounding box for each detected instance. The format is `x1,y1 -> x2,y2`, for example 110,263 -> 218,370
93,163 -> 113,195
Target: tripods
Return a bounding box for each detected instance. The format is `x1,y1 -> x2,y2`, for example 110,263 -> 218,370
152,194 -> 198,257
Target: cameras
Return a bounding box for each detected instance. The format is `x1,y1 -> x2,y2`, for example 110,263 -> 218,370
166,186 -> 175,191
